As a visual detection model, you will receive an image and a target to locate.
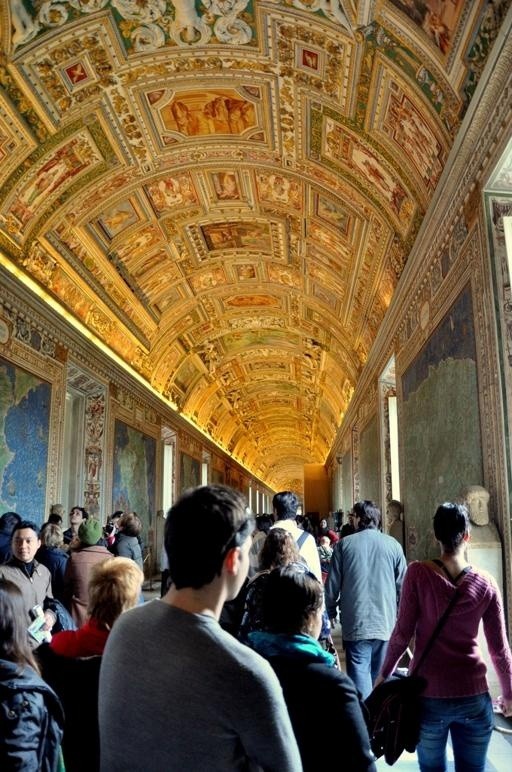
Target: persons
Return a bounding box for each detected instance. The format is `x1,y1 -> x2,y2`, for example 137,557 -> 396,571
1,482 -> 512,771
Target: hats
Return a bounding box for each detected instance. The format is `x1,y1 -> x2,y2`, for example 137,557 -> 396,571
78,516 -> 105,546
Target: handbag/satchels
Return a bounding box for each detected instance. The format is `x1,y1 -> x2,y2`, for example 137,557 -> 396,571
359,673 -> 431,769
